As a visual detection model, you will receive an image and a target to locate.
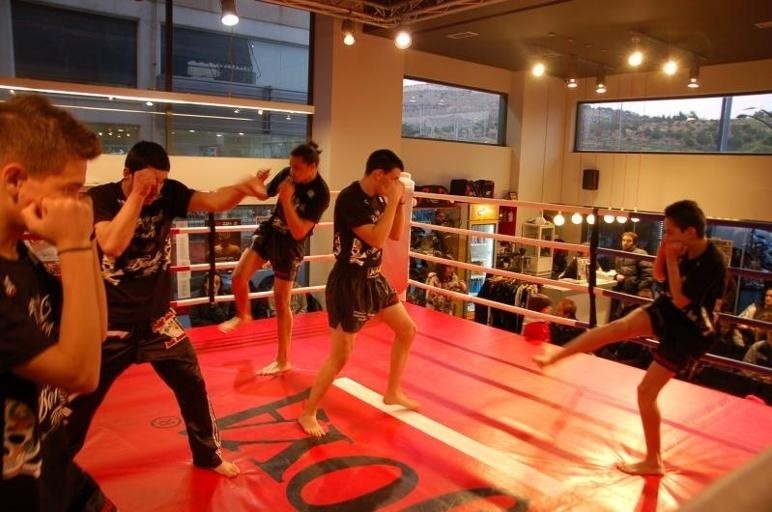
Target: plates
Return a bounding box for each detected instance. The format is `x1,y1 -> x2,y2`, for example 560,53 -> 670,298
521,222 -> 556,276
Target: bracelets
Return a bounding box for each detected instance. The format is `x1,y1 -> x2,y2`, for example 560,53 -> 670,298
57,246 -> 93,256
90,229 -> 96,240
399,202 -> 406,204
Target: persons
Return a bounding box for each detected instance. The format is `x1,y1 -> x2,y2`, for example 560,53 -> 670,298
194,272 -> 230,323
609,232 -> 770,400
424,255 -> 468,316
268,277 -> 308,316
563,241 -> 603,280
550,299 -> 588,347
531,201 -> 725,474
218,142 -> 330,375
522,294 -> 553,343
297,150 -> 419,437
0,93 -> 118,511
61,141 -> 271,477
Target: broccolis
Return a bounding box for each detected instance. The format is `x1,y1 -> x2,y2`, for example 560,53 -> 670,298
446,201 -> 497,320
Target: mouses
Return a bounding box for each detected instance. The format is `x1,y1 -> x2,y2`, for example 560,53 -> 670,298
488,272 -> 539,296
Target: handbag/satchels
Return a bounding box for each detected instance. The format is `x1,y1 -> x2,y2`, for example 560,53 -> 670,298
90,229 -> 96,240
399,202 -> 406,204
57,246 -> 93,256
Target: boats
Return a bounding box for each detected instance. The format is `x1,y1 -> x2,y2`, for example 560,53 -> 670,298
582,169 -> 598,190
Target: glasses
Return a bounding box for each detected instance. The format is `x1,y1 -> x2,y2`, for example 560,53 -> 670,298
525,42 -> 615,95
216,0 -> 509,51
553,203 -> 642,227
625,29 -> 711,90
